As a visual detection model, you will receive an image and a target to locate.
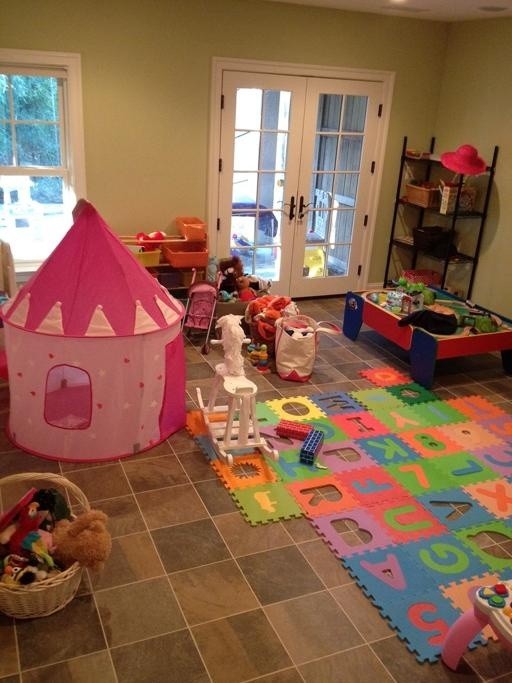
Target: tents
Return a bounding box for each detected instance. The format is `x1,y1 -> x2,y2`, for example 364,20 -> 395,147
0,197 -> 190,464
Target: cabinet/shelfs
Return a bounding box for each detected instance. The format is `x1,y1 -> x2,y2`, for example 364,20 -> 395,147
383,136 -> 499,301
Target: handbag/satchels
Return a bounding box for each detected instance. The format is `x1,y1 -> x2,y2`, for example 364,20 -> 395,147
275,314 -> 342,383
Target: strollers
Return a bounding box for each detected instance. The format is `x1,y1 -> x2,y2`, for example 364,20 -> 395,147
182,268 -> 223,353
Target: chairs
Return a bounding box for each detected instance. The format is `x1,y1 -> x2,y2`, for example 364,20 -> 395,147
305,192 -> 332,277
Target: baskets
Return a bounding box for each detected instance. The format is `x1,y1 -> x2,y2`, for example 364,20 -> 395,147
1,472 -> 91,620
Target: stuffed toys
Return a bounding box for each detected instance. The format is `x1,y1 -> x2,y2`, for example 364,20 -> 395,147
217,256 -> 271,302
248,293 -> 315,340
0,484 -> 112,590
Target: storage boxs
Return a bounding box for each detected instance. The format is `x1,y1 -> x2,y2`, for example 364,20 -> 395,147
119,216 -> 209,301
216,291 -> 270,315
414,226 -> 457,251
406,183 -> 440,208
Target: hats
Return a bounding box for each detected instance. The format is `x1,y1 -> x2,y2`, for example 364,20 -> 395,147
441,144 -> 487,175
